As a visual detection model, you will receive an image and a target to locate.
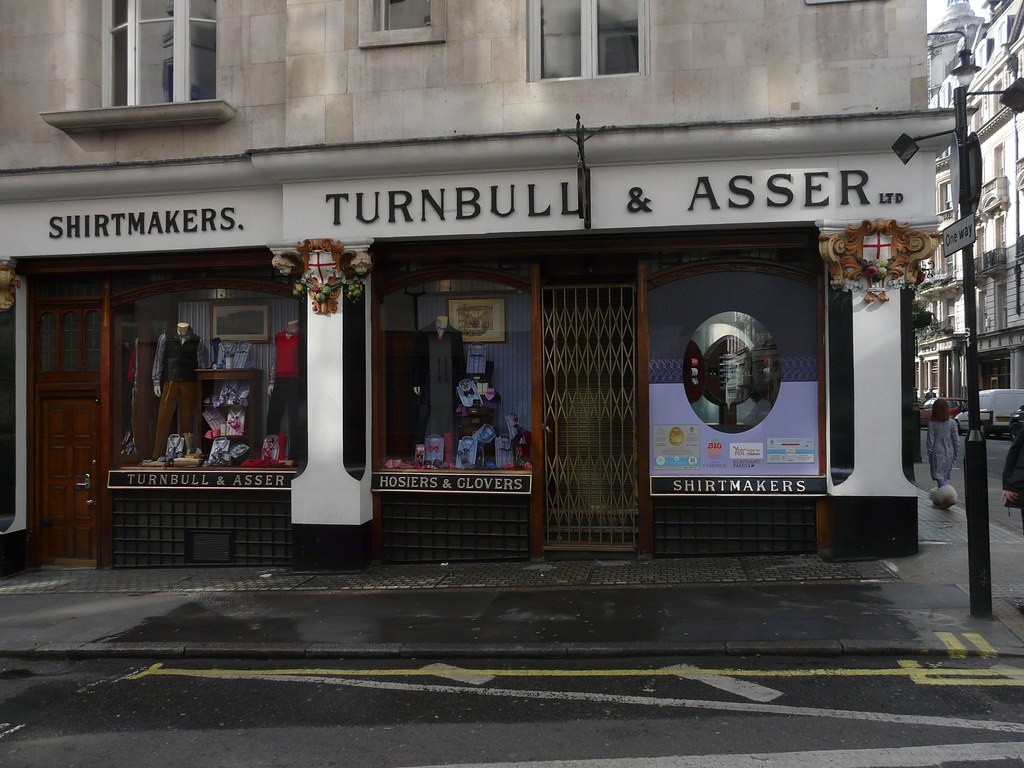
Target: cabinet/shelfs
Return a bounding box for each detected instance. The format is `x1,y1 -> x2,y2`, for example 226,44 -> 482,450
193,367 -> 263,455
457,391 -> 501,463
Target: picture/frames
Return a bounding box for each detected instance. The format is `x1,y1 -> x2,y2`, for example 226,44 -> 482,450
209,302 -> 272,344
448,298 -> 507,343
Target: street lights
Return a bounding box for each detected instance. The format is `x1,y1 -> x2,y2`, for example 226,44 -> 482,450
945,78 -> 1024,619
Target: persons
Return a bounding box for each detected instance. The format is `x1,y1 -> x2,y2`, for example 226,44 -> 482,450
925,399 -> 960,508
1000,428 -> 1024,617
350,252 -> 372,277
271,255 -> 295,276
408,315 -> 465,458
264,319 -> 299,463
145,322 -> 208,462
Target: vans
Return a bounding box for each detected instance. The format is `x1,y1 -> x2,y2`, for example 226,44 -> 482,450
954,389 -> 1024,438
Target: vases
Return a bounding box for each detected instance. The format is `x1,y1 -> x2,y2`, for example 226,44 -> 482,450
913,311 -> 932,327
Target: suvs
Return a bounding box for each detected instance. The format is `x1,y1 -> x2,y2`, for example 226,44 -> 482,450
1008,404 -> 1024,443
919,397 -> 968,428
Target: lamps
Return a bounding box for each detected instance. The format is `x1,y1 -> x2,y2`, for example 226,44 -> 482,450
928,31 -> 982,88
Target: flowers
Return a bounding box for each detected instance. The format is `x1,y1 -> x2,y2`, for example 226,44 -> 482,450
912,296 -> 931,314
856,257 -> 889,282
